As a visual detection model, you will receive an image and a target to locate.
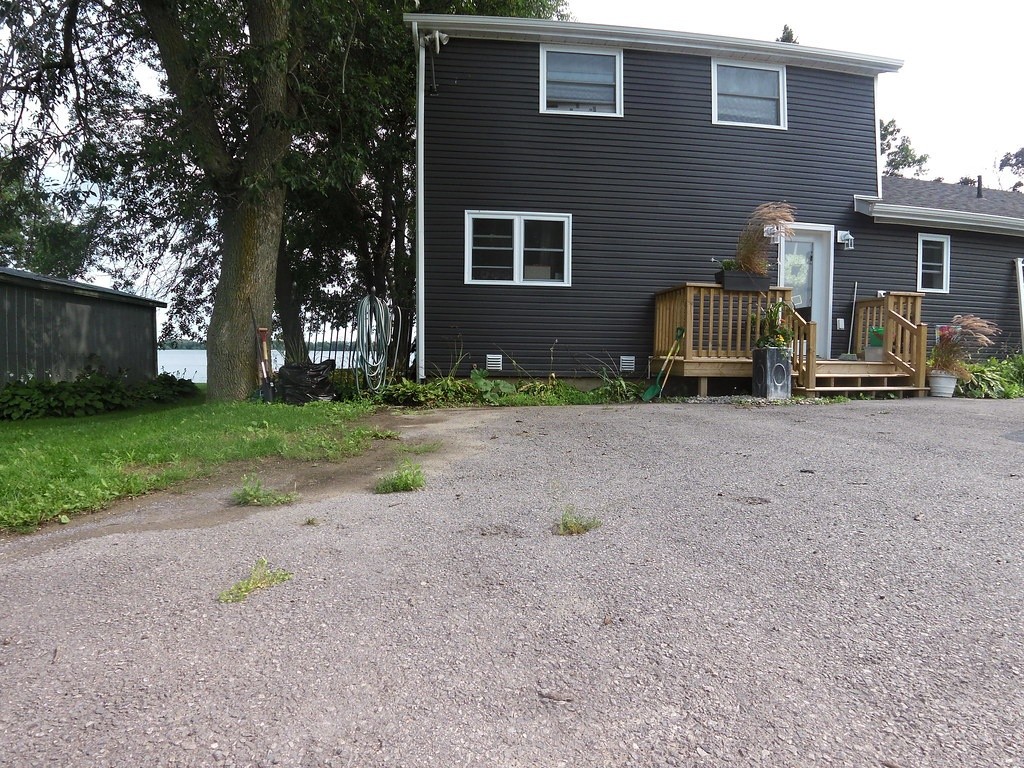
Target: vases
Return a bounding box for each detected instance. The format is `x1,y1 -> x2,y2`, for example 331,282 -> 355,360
928,369 -> 957,397
753,347 -> 791,400
715,271 -> 771,292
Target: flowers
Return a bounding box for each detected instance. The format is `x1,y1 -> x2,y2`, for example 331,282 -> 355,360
720,200 -> 798,271
929,314 -> 1002,378
749,300 -> 801,348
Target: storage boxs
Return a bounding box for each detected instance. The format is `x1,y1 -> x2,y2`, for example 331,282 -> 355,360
864,347 -> 883,362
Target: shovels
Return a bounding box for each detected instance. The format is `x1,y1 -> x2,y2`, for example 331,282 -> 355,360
248,296 -> 276,403
642,327 -> 685,403
257,327 -> 271,385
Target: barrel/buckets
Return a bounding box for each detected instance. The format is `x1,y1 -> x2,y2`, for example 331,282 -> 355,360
869,326 -> 884,346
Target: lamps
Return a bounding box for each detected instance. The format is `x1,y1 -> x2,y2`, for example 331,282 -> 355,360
763,224 -> 780,243
425,30 -> 449,45
837,230 -> 854,251
